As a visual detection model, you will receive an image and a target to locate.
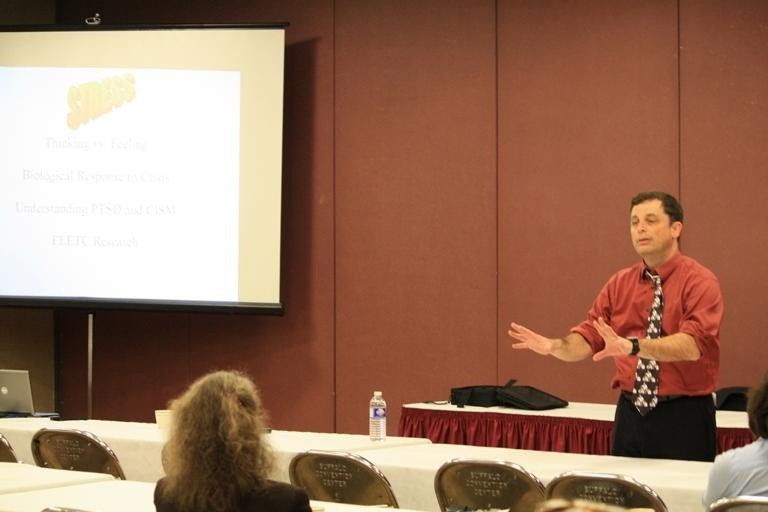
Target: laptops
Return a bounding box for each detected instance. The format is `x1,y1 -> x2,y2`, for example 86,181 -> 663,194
0,370 -> 61,418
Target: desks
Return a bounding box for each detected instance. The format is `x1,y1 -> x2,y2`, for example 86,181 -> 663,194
0,402 -> 751,512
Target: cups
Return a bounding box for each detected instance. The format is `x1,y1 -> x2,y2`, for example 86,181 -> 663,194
154,409 -> 173,437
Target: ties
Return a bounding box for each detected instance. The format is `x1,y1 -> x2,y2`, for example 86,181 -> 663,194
632,270 -> 663,417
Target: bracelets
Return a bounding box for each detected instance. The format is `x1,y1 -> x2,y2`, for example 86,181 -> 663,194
626,336 -> 640,357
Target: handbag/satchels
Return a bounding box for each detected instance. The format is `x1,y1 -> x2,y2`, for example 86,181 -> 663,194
451,385 -> 568,410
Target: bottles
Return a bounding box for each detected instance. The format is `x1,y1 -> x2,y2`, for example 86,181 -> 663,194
369,390 -> 387,440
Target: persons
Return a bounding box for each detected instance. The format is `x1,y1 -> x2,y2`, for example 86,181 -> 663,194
702,377 -> 768,511
152,369 -> 311,511
505,191 -> 726,461
